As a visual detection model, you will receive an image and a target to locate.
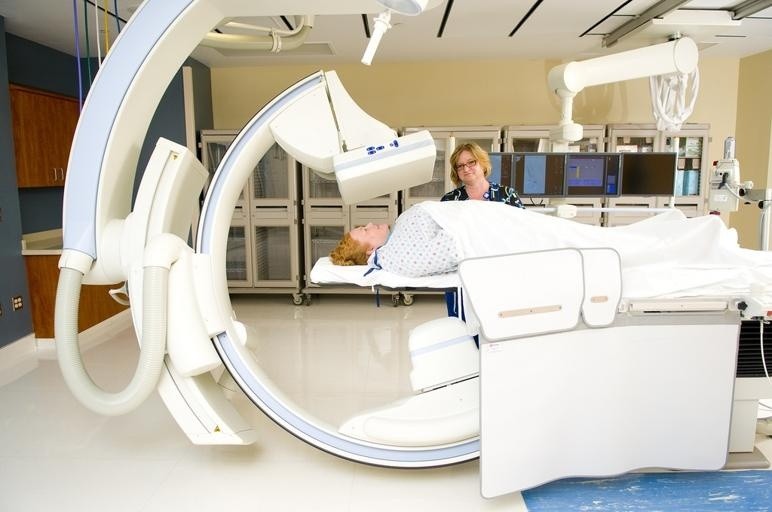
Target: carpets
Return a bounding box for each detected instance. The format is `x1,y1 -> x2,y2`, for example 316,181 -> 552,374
515,457 -> 772,511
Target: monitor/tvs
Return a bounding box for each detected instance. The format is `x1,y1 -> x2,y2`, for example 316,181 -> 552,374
487,151 -> 678,198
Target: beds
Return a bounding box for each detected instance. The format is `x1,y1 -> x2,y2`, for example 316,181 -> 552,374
311,234 -> 772,327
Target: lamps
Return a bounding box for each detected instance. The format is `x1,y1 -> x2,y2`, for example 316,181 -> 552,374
546,37 -> 699,143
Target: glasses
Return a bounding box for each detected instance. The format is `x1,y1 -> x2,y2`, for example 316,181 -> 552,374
454,160 -> 478,170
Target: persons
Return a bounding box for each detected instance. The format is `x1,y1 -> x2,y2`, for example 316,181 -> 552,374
328,197 -> 460,278
439,143 -> 526,349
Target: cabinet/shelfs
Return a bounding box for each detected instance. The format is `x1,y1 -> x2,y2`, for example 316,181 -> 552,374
401,126 -> 502,307
505,126 -> 607,227
201,129 -> 303,304
608,124 -> 710,227
303,166 -> 400,306
